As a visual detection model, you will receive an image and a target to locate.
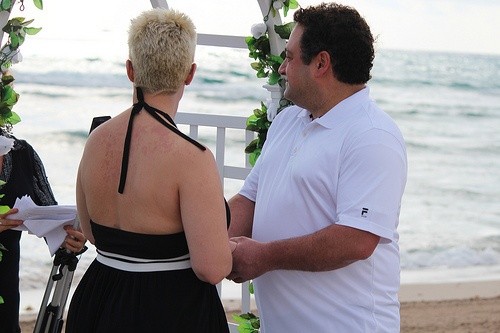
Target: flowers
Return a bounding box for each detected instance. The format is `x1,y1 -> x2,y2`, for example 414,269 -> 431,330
250,23 -> 267,39
0,44 -> 23,72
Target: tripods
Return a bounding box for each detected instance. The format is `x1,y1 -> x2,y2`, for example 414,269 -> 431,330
33,115 -> 112,333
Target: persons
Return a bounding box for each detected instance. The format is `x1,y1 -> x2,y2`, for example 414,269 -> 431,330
0,126 -> 87,333
63,5 -> 236,333
227,3 -> 408,332
